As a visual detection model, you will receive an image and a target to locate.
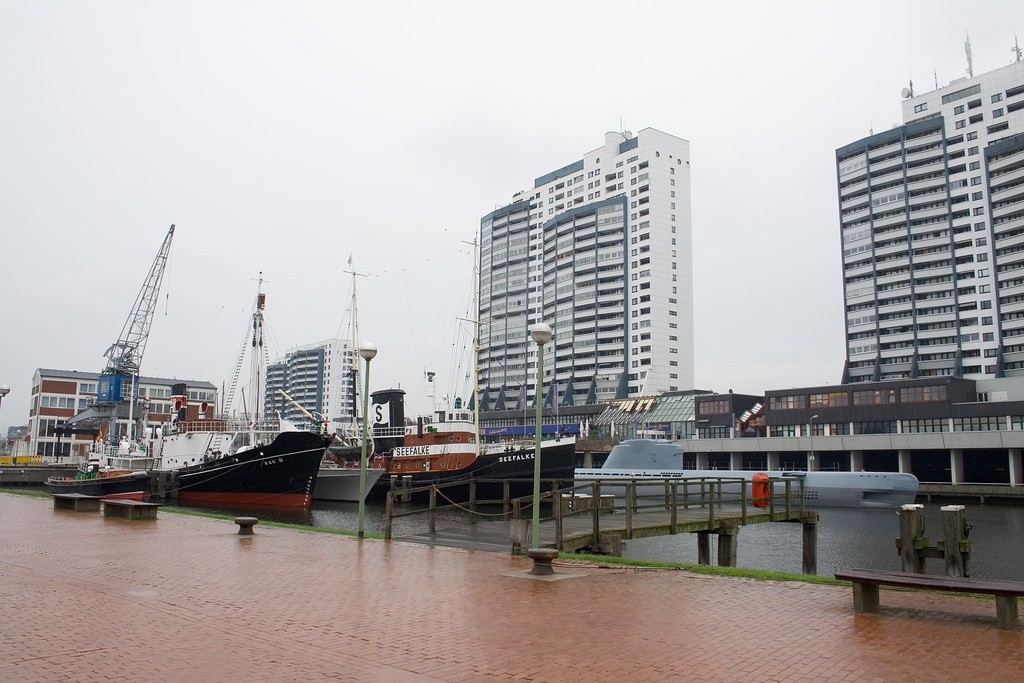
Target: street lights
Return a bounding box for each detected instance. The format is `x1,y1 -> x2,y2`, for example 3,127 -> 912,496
357,344 -> 378,540
0,384 -> 11,409
528,322 -> 554,552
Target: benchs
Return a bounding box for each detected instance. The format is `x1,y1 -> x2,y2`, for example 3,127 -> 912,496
50,493 -> 106,512
832,568 -> 1024,629
99,499 -> 162,521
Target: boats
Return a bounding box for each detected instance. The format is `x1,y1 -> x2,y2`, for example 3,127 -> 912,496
157,430 -> 387,504
573,437 -> 921,509
367,389 -> 580,507
146,431 -> 339,505
45,468 -> 152,496
